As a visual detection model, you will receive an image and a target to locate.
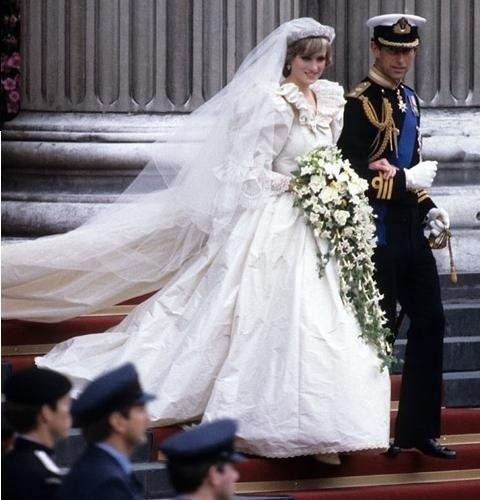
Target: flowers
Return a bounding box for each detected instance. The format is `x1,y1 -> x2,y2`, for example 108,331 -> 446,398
286,140 -> 400,370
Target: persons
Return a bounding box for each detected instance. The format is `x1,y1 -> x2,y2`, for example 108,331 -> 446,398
2,362 -> 246,499
348,12 -> 466,461
211,24 -> 377,465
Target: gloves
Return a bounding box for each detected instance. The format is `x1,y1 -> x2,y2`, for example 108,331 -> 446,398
403,160 -> 437,191
423,207 -> 449,239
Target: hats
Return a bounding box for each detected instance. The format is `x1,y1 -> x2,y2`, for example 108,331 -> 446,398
366,13 -> 427,49
2,362 -> 251,467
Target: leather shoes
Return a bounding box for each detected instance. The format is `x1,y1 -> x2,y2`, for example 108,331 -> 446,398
394,429 -> 457,459
